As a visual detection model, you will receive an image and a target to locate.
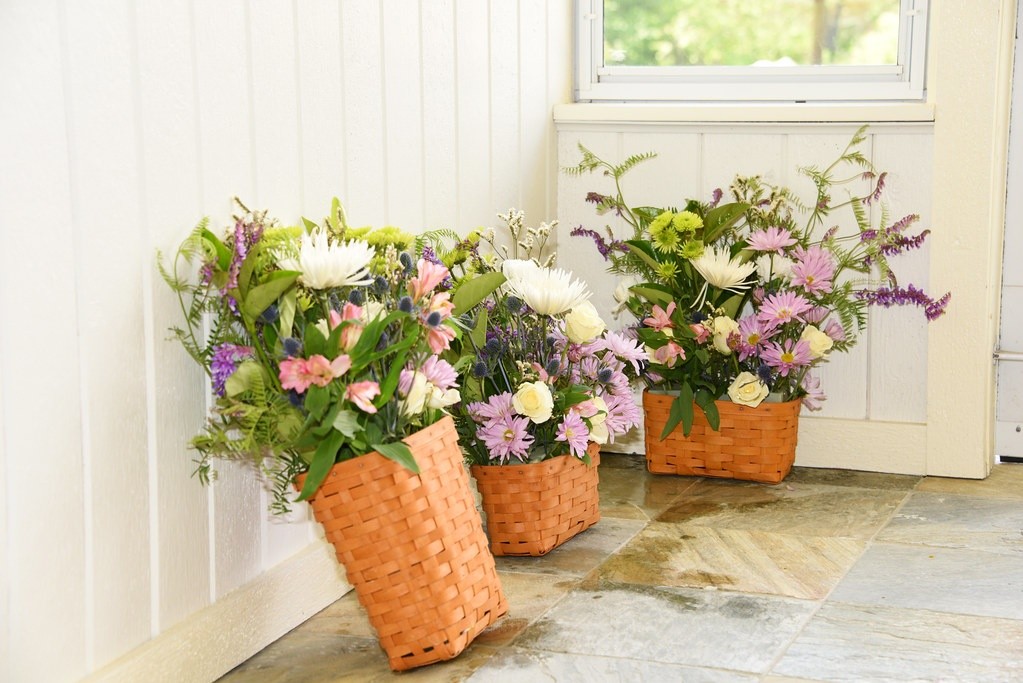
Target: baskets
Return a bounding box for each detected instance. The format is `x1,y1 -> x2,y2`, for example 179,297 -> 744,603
471,439 -> 603,556
292,416 -> 509,672
642,379 -> 801,484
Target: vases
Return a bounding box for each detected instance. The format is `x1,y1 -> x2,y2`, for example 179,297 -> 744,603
291,390 -> 802,671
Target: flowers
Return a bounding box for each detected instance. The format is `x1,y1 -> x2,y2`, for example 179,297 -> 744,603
156,121 -> 953,523
